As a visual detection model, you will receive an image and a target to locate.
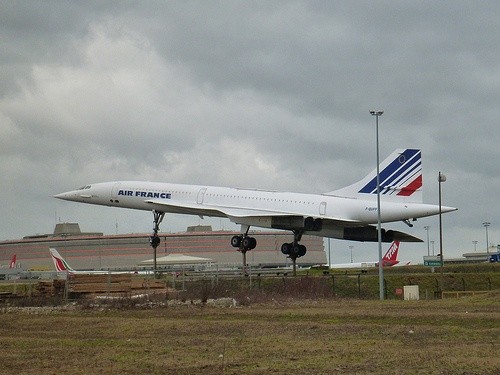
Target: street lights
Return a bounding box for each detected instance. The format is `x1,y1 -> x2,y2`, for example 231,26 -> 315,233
472,240 -> 479,253
436,171 -> 446,266
368,110 -> 385,300
482,221 -> 492,262
423,225 -> 432,256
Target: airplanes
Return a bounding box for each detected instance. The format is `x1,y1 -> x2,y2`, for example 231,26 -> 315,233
48,247 -> 183,283
310,241 -> 400,271
51,147 -> 458,259
